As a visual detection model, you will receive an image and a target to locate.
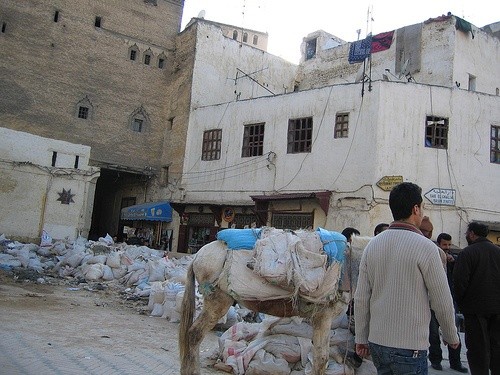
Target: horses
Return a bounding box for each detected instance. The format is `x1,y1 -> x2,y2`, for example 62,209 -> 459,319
178,226 -> 446,375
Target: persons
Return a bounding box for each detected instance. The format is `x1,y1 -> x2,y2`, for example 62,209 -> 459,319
452,222 -> 500,375
419,216 -> 468,373
354,182 -> 460,375
341,223 -> 390,368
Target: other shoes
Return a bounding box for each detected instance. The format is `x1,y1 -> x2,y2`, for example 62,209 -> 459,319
432,361 -> 442,370
339,345 -> 362,370
450,365 -> 468,373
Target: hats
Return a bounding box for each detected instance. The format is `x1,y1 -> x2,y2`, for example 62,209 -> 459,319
420,216 -> 433,232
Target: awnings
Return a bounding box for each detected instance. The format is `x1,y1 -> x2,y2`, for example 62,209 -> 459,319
121,201 -> 172,223
